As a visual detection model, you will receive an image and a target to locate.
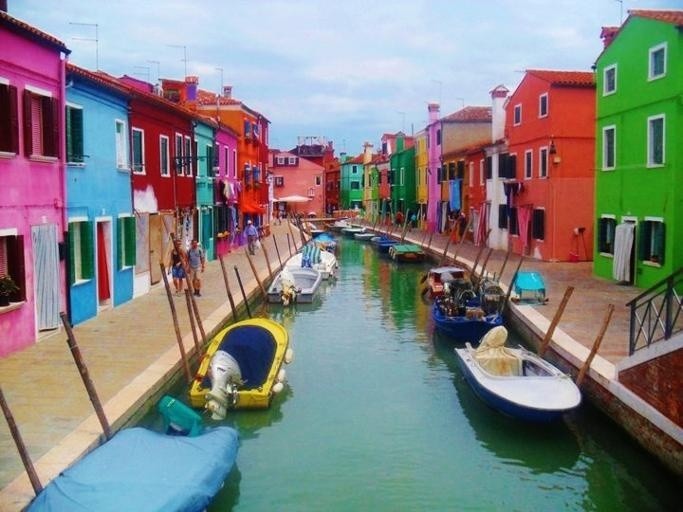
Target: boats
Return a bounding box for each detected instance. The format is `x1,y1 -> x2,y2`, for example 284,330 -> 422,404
266,213 -> 505,342
187,318 -> 293,421
452,326 -> 585,425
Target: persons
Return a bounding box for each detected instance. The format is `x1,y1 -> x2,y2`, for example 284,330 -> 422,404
455,211 -> 467,243
243,220 -> 259,255
272,208 -> 283,226
168,239 -> 204,297
395,209 -> 417,232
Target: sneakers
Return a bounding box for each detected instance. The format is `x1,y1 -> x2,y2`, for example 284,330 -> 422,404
176,290 -> 201,296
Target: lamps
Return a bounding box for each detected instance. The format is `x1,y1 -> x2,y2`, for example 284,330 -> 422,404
173,154 -> 220,173
